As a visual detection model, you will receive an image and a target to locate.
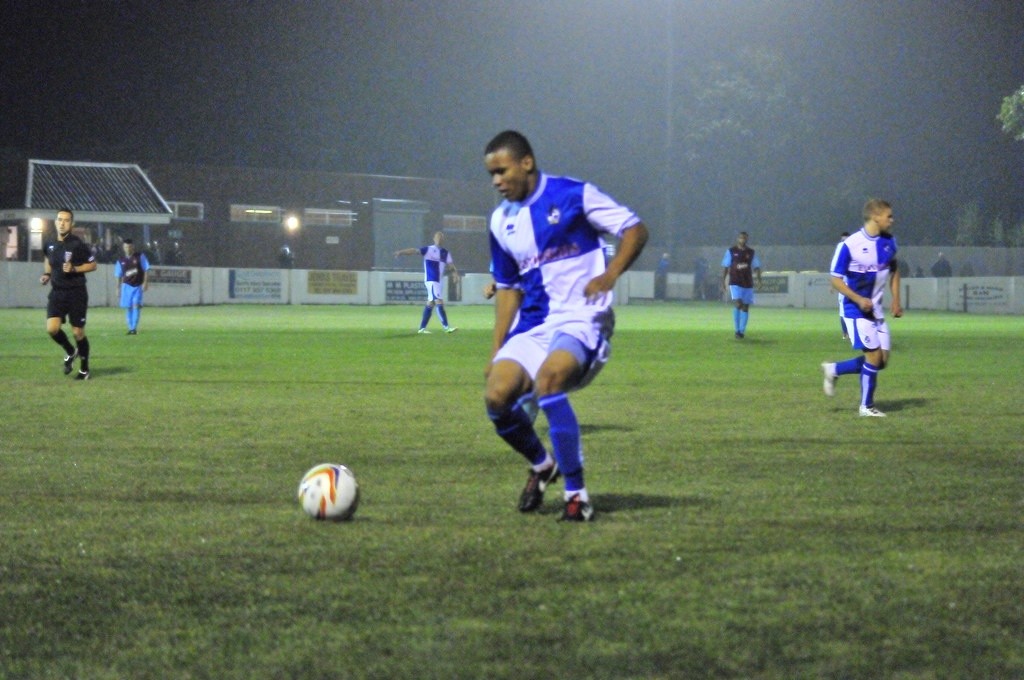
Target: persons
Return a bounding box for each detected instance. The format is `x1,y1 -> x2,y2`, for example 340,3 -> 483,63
39,208 -> 98,379
482,131 -> 649,521
836,231 -> 850,340
392,231 -> 460,334
693,251 -> 712,300
820,197 -> 902,417
896,250 -> 952,278
114,239 -> 151,335
721,231 -> 763,340
653,253 -> 671,301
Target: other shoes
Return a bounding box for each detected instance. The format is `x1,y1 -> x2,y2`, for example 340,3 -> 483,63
734,331 -> 744,339
444,327 -> 458,333
127,330 -> 137,335
75,370 -> 90,381
418,328 -> 431,334
63,347 -> 79,375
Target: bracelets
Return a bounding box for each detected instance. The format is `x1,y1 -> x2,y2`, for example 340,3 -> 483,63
44,273 -> 51,277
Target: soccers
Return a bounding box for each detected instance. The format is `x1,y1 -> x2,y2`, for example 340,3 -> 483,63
297,462 -> 360,520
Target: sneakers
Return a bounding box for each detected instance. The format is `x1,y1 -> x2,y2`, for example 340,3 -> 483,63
518,460 -> 560,513
859,404 -> 887,417
821,362 -> 838,397
555,493 -> 595,524
